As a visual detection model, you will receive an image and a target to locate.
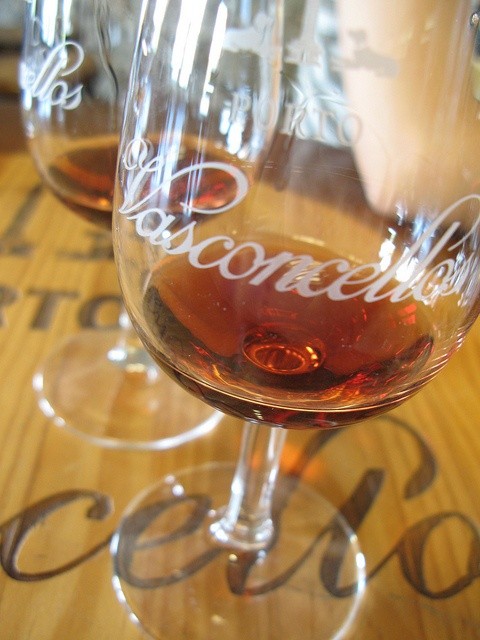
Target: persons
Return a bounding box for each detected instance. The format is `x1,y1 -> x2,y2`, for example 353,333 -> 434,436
299,0 -> 480,233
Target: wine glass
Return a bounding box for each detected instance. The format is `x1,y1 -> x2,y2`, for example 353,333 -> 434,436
14,1 -> 289,454
104,0 -> 478,640
